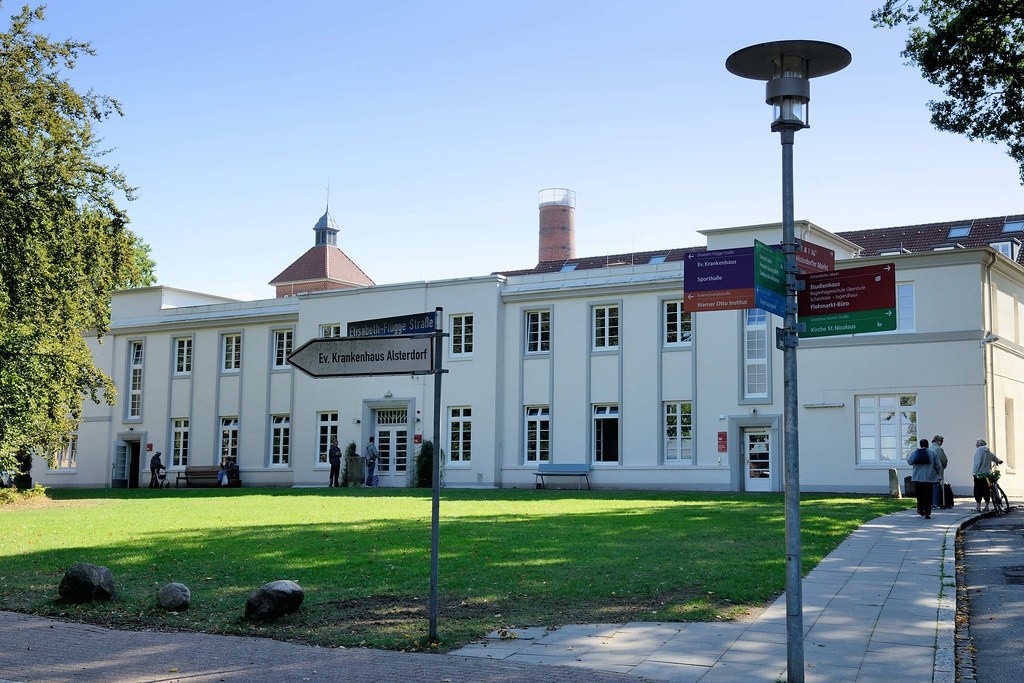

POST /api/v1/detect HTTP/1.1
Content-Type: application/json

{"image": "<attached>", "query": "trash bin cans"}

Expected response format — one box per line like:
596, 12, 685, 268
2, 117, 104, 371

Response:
904, 476, 917, 497
347, 457, 366, 481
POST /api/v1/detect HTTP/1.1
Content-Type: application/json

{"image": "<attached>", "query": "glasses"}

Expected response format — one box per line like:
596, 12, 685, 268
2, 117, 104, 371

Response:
937, 439, 943, 442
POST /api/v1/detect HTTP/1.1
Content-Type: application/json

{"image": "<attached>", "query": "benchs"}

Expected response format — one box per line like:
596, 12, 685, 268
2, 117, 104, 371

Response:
176, 465, 242, 488
532, 464, 591, 491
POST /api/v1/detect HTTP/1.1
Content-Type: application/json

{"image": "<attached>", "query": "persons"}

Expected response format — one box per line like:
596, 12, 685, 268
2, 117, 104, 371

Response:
329, 440, 342, 488
364, 436, 383, 487
217, 455, 236, 483
929, 435, 948, 510
907, 439, 940, 519
148, 452, 164, 488
971, 439, 1001, 512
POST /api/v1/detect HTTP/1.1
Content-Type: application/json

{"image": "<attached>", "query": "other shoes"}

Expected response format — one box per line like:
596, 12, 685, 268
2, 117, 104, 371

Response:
334, 485, 340, 487
329, 485, 334, 488
971, 508, 981, 512
917, 510, 931, 520
982, 508, 989, 511
364, 484, 372, 488
931, 504, 940, 509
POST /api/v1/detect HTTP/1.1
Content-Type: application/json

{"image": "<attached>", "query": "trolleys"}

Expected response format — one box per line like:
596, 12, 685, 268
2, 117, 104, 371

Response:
153, 467, 169, 488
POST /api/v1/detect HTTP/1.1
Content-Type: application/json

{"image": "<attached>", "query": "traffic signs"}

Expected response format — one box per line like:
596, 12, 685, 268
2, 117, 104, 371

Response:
286, 338, 434, 380
683, 238, 897, 338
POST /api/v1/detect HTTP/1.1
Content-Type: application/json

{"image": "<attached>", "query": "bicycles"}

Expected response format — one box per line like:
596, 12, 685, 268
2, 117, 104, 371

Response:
987, 462, 1009, 516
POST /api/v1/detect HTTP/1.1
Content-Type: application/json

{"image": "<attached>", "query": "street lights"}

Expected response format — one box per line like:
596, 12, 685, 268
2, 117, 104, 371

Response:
724, 40, 854, 683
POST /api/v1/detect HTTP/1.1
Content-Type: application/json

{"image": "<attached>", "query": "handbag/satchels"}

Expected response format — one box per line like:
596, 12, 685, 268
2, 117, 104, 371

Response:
914, 447, 932, 465
221, 473, 228, 486
369, 457, 375, 464
